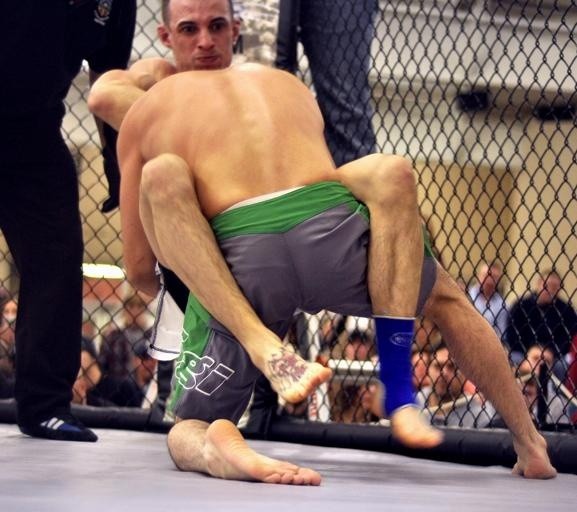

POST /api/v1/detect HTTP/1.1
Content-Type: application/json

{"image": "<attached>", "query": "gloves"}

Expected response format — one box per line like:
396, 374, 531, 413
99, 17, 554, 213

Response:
101, 159, 121, 212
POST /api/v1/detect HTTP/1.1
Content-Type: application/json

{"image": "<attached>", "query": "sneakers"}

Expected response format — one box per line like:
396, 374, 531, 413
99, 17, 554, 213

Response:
20, 414, 98, 442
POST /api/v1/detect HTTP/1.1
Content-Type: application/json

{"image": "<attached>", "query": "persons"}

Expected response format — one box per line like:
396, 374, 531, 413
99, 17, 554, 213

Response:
0, 296, 18, 383
294, 2, 379, 168
87, 1, 443, 448
411, 262, 576, 431
0, 1, 136, 442
116, 66, 556, 489
250, 309, 381, 424
72, 292, 156, 408
